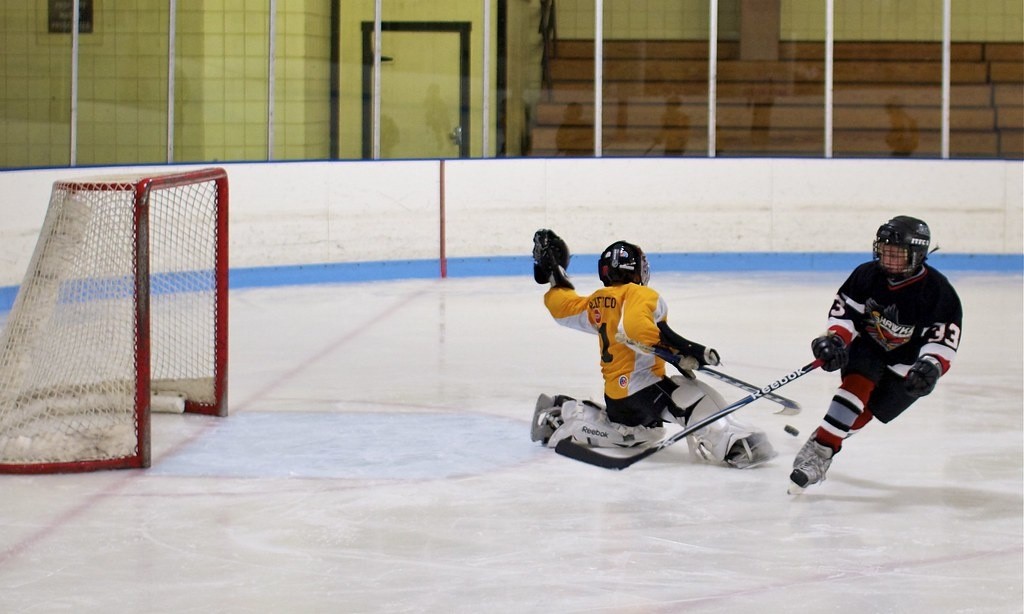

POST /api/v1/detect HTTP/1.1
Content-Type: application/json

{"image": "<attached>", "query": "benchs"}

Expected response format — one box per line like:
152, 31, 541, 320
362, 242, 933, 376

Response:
523, 39, 1024, 159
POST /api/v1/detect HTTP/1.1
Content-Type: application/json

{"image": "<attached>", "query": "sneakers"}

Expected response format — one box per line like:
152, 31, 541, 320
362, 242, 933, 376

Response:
786, 430, 850, 495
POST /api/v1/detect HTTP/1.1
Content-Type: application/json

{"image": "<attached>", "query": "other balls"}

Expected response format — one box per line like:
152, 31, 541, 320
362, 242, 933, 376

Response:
783, 423, 800, 436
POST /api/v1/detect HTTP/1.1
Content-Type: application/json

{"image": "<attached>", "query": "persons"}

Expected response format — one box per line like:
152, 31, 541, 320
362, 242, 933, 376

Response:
786, 216, 963, 497
530, 229, 781, 471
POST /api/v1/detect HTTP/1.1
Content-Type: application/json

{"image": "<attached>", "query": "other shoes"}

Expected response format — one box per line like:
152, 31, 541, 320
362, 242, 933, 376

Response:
530, 393, 557, 443
724, 433, 779, 469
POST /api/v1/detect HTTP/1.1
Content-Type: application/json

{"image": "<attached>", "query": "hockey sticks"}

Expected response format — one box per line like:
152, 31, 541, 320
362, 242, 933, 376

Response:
613, 333, 802, 417
554, 358, 827, 472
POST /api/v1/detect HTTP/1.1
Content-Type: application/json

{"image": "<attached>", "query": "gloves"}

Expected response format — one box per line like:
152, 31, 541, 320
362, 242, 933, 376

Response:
811, 336, 849, 372
902, 354, 942, 397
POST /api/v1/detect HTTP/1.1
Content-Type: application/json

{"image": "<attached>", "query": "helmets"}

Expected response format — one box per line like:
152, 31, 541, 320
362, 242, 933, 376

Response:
598, 241, 641, 286
877, 215, 930, 265
